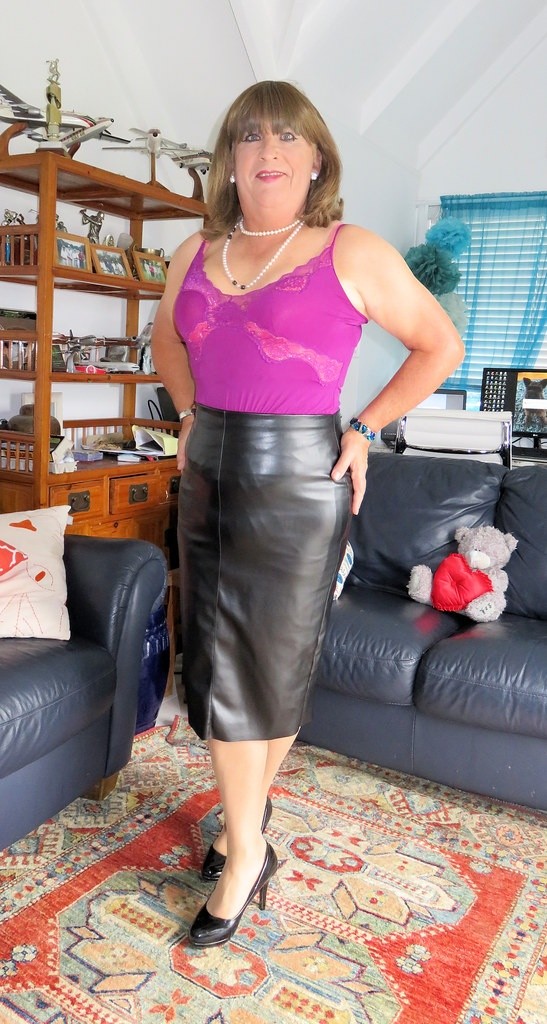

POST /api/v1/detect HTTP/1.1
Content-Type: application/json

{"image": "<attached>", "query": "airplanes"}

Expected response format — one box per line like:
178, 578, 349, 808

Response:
104, 321, 155, 348
129, 127, 214, 175
55, 330, 120, 352
0, 83, 131, 150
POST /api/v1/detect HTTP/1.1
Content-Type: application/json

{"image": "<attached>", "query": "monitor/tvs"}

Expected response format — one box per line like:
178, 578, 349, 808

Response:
416, 390, 467, 409
479, 367, 547, 458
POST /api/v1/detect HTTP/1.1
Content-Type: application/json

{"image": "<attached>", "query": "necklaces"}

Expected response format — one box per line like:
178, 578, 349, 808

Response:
222, 214, 306, 292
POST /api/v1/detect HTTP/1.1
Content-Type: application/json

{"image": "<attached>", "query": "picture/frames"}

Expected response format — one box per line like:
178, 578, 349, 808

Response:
130, 251, 167, 283
89, 245, 133, 277
53, 230, 92, 273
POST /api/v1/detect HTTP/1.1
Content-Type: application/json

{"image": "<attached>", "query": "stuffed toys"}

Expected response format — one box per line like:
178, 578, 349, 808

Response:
406, 526, 519, 622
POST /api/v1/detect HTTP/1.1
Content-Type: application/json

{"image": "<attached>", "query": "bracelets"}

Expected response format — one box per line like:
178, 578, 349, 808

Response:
179, 404, 197, 422
350, 418, 376, 443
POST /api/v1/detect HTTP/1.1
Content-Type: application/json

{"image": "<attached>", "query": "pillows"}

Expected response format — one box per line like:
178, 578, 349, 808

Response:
0, 505, 74, 640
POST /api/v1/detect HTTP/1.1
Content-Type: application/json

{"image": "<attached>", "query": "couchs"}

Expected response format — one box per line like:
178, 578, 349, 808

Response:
294, 452, 547, 811
0, 534, 167, 851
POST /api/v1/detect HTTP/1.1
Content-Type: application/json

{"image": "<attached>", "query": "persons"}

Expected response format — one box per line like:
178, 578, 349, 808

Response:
46, 58, 60, 82
1, 209, 26, 226
141, 260, 161, 280
56, 215, 67, 232
61, 245, 85, 268
151, 80, 465, 946
80, 209, 104, 244
97, 253, 126, 276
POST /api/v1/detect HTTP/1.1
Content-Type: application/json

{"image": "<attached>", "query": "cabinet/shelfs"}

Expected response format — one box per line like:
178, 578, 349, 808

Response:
0, 121, 213, 572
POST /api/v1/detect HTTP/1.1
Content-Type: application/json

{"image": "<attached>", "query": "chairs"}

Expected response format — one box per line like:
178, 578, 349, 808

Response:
393, 411, 513, 471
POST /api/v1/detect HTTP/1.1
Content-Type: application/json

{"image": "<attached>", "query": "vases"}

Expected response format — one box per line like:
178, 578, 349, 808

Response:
134, 605, 171, 734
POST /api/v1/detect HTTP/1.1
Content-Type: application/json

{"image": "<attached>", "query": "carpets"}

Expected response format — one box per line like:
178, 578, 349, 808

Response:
0, 715, 547, 1024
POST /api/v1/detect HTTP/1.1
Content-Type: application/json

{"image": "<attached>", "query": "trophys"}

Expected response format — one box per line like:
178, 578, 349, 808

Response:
132, 244, 165, 280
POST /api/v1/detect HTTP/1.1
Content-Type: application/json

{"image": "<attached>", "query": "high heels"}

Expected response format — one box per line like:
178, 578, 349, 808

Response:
201, 797, 272, 882
189, 844, 278, 947
